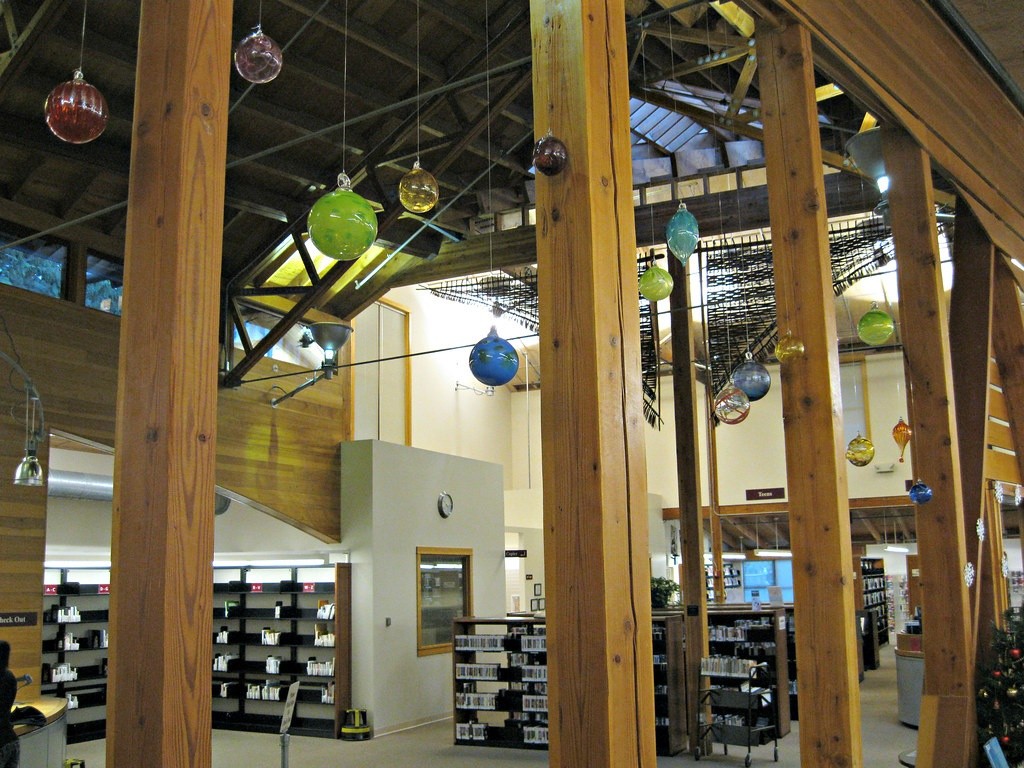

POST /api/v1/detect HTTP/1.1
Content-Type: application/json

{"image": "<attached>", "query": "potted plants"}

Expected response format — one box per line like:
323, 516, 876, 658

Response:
649, 576, 681, 607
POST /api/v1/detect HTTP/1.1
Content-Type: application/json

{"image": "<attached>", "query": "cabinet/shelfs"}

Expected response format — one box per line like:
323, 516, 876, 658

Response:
908, 555, 920, 617
212, 559, 347, 737
452, 615, 554, 751
42, 563, 110, 745
853, 560, 889, 646
651, 601, 799, 756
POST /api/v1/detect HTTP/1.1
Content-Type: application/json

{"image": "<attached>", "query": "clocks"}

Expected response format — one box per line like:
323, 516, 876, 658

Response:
437, 490, 454, 520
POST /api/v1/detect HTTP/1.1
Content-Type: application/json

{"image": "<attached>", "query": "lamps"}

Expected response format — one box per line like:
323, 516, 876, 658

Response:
454, 380, 495, 397
843, 126, 893, 226
1, 352, 47, 488
265, 323, 354, 409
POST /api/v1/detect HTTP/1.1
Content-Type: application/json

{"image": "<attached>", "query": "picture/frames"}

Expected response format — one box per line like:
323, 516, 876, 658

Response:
534, 583, 542, 596
538, 598, 545, 610
531, 599, 538, 610
